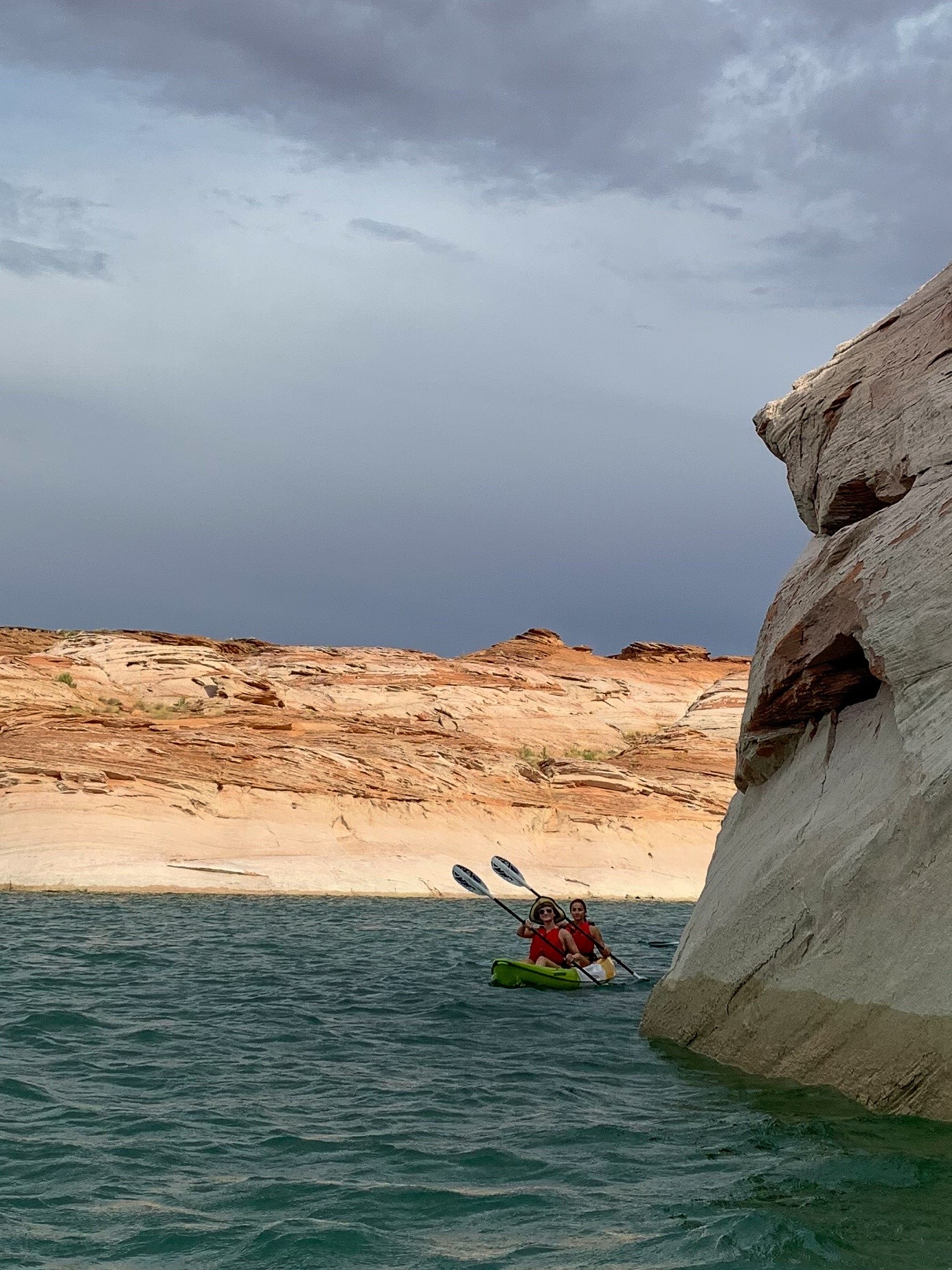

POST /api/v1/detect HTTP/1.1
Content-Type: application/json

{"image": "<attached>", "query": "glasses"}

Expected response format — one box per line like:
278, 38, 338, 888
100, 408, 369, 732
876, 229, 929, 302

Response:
539, 909, 552, 914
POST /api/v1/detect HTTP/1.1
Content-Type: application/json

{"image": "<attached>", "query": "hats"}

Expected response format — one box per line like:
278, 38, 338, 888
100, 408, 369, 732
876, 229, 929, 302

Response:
529, 896, 566, 925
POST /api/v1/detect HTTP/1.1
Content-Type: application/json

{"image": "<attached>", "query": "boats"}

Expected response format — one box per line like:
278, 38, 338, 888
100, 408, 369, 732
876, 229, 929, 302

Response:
491, 954, 617, 992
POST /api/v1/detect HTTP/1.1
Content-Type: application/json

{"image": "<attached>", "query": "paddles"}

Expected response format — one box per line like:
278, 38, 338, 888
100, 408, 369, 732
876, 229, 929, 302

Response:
637, 941, 680, 948
490, 856, 648, 980
452, 864, 606, 986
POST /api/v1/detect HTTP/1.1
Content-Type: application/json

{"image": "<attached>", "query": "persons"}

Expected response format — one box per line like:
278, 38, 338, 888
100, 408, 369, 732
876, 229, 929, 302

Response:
517, 897, 612, 968
